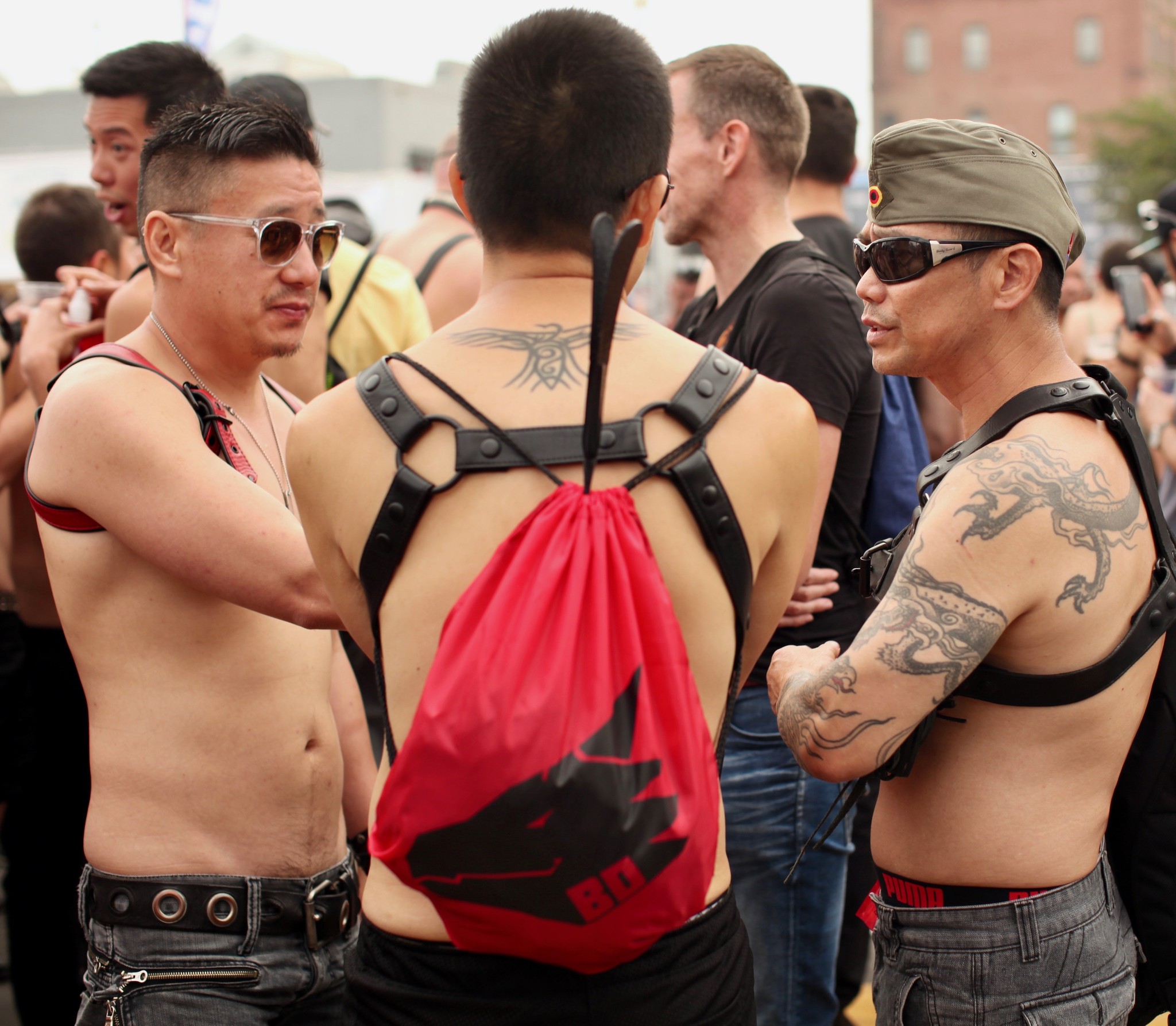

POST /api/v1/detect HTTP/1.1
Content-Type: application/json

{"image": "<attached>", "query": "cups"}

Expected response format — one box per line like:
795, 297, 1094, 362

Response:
14, 279, 77, 357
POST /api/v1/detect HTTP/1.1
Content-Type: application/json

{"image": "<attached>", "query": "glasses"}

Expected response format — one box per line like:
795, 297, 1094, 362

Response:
140, 212, 346, 271
621, 166, 675, 211
852, 236, 1043, 285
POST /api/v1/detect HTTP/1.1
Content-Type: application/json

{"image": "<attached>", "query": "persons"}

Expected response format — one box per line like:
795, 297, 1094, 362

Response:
0, 11, 1176, 1025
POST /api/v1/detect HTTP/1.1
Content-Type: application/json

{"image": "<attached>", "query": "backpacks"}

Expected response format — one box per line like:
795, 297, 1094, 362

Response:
368, 352, 761, 976
861, 374, 934, 551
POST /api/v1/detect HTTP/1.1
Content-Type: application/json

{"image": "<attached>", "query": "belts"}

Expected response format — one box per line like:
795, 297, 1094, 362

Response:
86, 844, 360, 954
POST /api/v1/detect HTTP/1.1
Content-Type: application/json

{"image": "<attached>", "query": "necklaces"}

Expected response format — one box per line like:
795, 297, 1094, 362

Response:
150, 314, 291, 510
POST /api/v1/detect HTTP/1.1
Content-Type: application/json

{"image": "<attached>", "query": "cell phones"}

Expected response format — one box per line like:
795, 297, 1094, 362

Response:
1111, 266, 1155, 335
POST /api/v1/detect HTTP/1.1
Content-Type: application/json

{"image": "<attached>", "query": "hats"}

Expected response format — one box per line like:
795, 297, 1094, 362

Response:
866, 118, 1086, 280
